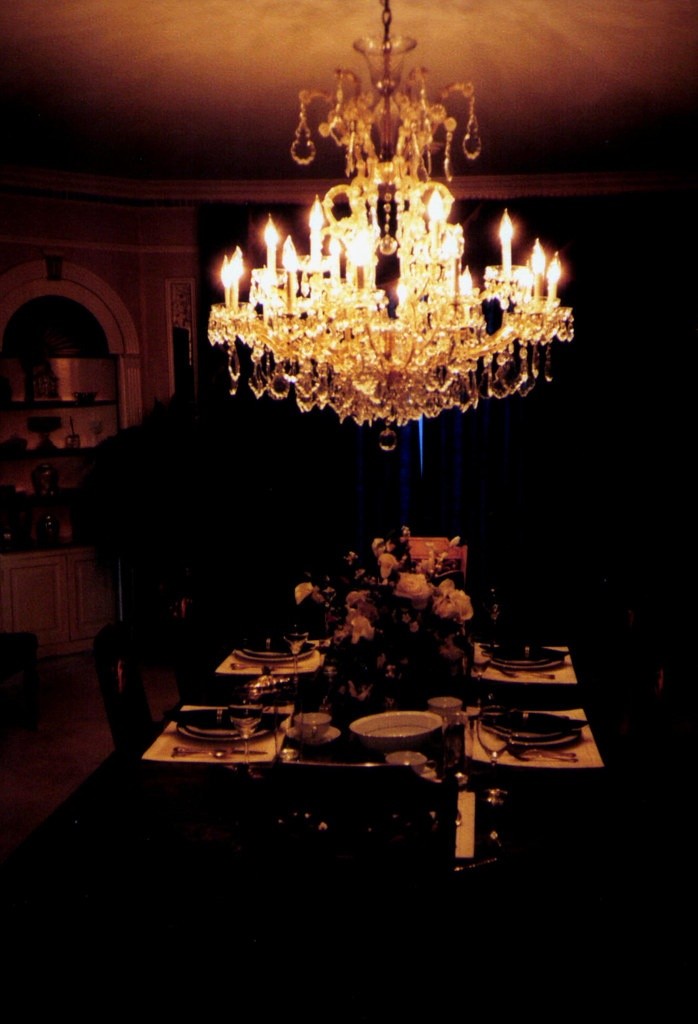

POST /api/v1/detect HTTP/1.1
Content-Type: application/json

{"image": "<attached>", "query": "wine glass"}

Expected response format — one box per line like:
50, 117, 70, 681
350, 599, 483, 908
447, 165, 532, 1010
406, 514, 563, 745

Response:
478, 694, 515, 803
281, 604, 309, 694
228, 685, 263, 766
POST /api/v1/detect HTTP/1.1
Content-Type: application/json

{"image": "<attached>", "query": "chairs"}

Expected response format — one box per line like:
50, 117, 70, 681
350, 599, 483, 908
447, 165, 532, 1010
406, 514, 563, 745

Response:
94, 602, 221, 744
581, 602, 668, 829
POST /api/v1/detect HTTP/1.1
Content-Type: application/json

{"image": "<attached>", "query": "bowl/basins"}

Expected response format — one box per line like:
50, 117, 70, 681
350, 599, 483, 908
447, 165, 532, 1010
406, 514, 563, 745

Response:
349, 712, 443, 751
73, 392, 96, 401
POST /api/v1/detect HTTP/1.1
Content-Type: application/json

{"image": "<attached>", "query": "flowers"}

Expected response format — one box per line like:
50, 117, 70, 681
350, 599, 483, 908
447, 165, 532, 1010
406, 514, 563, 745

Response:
297, 525, 473, 701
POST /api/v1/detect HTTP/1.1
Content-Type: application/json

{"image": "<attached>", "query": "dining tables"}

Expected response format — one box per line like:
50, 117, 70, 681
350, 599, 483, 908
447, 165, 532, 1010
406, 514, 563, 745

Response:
140, 641, 615, 888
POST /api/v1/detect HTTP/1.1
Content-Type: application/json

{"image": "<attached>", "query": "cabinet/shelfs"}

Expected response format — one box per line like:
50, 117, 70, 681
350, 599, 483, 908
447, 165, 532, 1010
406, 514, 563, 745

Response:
0, 346, 118, 661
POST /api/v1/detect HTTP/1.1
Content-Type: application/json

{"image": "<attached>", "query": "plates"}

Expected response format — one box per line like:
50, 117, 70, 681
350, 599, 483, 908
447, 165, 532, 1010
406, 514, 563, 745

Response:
284, 726, 341, 743
480, 718, 581, 746
176, 708, 269, 741
489, 651, 564, 669
233, 641, 315, 662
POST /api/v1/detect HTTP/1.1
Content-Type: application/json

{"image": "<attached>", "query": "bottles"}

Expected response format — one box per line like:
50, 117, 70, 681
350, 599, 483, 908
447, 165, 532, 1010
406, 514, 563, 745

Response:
424, 696, 463, 767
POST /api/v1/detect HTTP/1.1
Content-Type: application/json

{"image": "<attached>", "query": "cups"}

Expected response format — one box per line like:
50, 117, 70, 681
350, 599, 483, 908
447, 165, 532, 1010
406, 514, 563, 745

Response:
386, 751, 437, 775
294, 712, 332, 736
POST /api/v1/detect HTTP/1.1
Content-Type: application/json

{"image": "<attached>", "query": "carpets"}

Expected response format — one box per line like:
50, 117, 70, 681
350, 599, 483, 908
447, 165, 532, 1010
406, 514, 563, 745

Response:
0, 721, 698, 1024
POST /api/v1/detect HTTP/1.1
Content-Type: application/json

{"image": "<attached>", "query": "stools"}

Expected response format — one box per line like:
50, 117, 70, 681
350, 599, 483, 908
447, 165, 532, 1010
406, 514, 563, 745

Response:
0, 629, 38, 742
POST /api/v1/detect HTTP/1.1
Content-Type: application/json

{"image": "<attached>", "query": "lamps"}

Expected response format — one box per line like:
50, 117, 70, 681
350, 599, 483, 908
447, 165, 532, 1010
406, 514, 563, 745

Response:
207, 0, 575, 452
